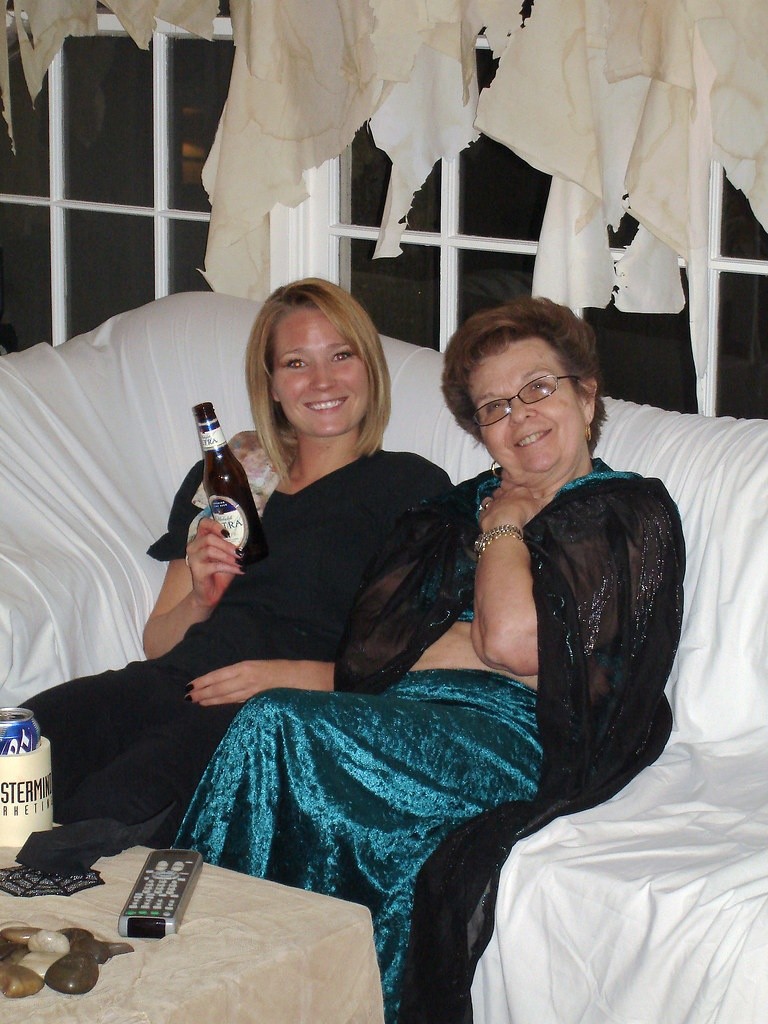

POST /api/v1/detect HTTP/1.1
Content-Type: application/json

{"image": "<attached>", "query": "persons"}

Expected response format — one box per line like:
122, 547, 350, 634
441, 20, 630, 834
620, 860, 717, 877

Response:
0, 278, 455, 849
172, 298, 686, 1024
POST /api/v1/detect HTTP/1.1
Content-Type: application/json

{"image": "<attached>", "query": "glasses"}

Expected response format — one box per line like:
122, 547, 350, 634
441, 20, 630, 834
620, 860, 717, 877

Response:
473, 374, 581, 426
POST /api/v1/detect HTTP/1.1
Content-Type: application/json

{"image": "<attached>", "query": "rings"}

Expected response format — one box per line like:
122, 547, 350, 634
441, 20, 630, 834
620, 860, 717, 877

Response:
484, 500, 491, 508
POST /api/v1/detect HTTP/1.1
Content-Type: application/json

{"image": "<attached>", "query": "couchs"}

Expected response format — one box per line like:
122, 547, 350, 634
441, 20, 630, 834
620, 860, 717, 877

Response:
0, 293, 767, 1024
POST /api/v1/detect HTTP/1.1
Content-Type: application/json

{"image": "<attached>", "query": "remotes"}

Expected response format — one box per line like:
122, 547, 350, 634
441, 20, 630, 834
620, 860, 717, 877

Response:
117, 848, 203, 940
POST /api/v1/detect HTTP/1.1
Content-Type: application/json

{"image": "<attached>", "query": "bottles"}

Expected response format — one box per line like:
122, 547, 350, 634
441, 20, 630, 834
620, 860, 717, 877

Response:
192, 402, 271, 568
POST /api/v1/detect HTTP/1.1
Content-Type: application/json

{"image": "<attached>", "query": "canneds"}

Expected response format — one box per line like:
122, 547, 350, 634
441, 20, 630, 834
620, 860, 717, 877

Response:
0, 707, 41, 754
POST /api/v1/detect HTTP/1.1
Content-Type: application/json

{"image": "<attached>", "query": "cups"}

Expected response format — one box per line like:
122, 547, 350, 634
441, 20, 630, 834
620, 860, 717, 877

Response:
0, 736, 52, 848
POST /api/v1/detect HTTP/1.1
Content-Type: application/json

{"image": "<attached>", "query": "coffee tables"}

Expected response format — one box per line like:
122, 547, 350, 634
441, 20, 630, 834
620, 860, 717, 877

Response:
0, 821, 386, 1024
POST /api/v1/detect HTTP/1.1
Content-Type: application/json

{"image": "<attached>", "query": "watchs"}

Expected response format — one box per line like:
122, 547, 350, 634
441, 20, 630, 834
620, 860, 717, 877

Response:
473, 524, 522, 558
